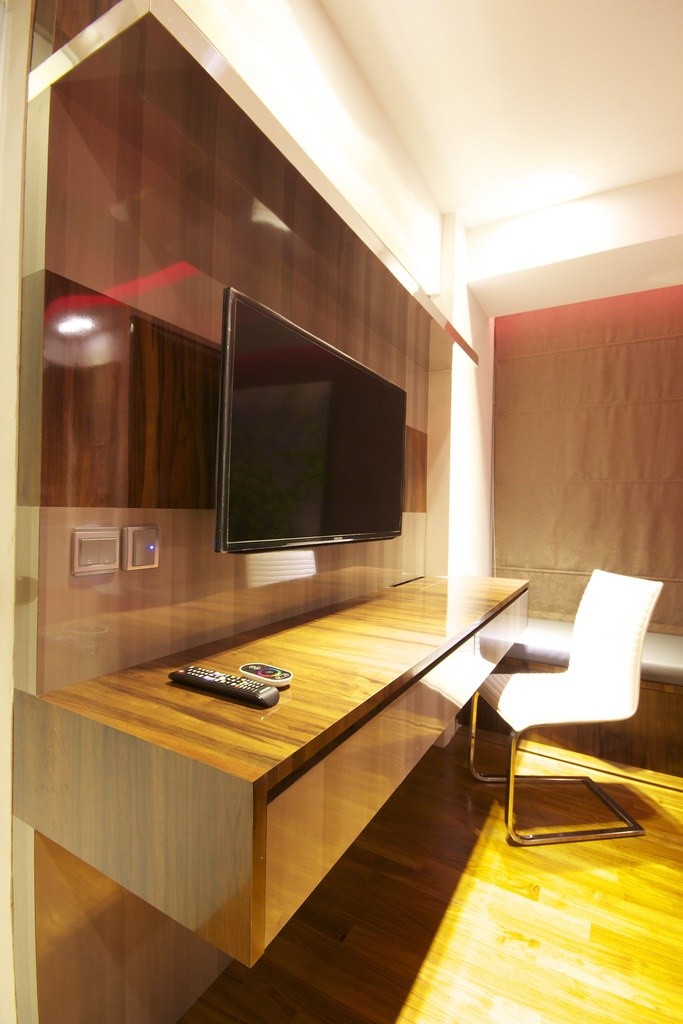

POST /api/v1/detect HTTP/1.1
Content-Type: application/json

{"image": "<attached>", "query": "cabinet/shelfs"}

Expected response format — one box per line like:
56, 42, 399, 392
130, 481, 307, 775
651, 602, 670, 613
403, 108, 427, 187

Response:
28, 569, 533, 969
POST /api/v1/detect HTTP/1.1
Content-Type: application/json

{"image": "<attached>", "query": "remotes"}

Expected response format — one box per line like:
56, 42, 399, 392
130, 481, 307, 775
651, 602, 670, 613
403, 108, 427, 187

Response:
169, 665, 280, 708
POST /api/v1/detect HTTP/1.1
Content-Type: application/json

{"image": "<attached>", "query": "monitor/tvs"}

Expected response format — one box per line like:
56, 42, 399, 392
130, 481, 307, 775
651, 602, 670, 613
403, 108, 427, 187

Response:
215, 287, 408, 553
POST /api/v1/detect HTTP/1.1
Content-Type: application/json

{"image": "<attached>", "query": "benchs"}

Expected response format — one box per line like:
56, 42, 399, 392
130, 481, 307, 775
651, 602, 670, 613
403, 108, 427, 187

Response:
460, 604, 683, 775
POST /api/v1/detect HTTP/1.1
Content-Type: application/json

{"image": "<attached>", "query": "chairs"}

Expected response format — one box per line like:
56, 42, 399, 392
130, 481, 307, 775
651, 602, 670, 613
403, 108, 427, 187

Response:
467, 570, 664, 844
